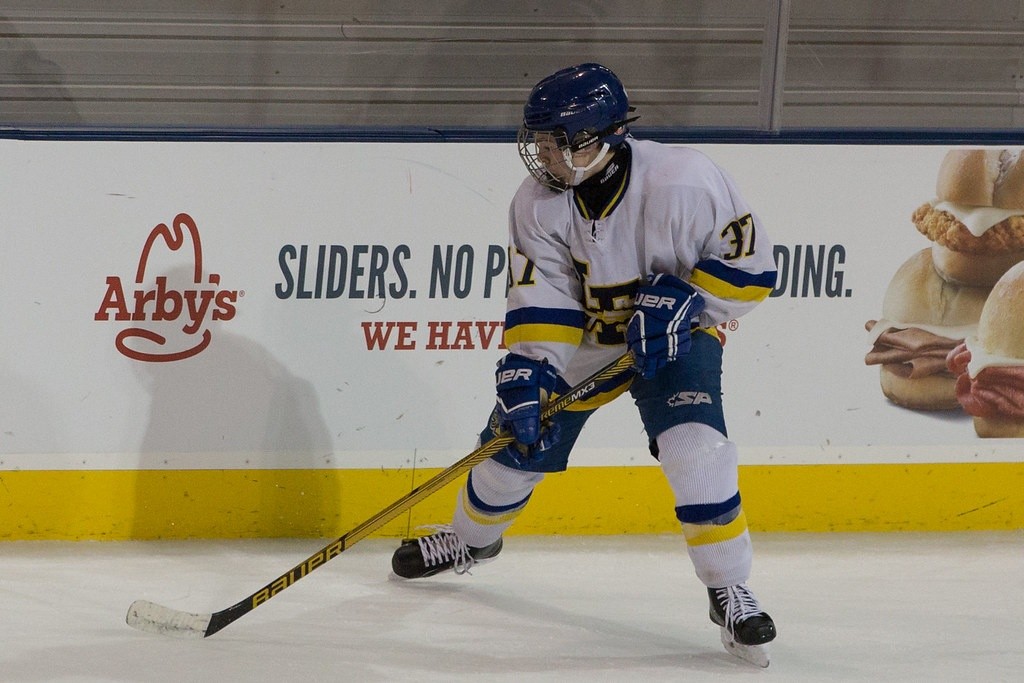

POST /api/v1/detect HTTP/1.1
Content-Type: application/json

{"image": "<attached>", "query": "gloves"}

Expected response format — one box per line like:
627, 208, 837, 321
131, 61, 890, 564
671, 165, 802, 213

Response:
624, 273, 705, 379
486, 352, 558, 464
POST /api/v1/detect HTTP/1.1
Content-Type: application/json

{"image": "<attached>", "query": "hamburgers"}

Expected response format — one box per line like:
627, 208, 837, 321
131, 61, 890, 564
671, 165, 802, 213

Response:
864, 144, 1024, 438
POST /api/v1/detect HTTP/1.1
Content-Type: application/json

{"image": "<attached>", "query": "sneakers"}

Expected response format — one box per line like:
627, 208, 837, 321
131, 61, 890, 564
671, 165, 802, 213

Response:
706, 583, 777, 667
389, 530, 503, 583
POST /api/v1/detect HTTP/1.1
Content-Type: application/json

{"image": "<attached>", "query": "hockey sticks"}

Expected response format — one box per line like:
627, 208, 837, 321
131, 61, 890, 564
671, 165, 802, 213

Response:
127, 320, 703, 639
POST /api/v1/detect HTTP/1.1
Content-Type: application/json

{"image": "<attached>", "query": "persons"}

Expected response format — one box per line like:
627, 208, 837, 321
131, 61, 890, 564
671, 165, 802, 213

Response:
392, 63, 778, 668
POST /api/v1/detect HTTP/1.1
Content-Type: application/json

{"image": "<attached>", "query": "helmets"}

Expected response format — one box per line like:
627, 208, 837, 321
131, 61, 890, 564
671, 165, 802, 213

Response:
524, 63, 630, 151
